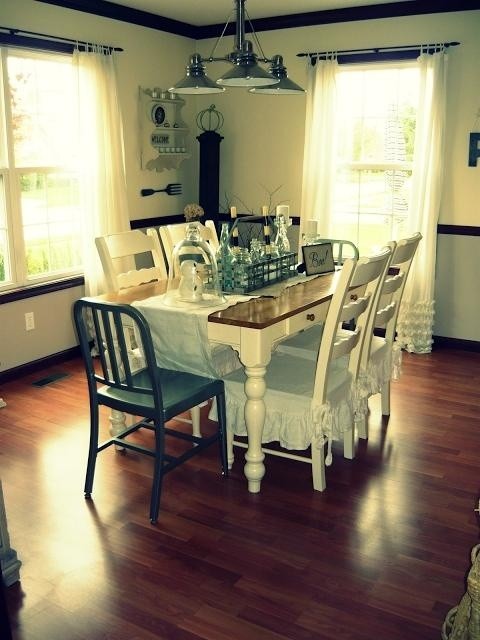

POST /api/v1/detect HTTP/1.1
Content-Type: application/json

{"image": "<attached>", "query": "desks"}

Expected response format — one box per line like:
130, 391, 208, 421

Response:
83, 256, 389, 493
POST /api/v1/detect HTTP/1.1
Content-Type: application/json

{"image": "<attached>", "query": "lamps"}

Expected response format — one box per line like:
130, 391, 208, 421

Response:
167, 0, 307, 95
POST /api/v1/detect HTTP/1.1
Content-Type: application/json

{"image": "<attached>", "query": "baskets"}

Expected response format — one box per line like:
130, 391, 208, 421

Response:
439, 544, 480, 640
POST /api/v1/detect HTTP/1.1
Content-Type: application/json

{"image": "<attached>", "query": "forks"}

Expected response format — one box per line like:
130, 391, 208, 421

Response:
141, 183, 182, 196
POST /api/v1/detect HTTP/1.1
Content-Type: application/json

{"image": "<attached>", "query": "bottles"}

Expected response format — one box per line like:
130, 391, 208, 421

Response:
213, 205, 290, 293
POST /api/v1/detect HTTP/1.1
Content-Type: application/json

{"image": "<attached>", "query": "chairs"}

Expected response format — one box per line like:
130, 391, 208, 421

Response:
70, 296, 230, 522
93, 227, 217, 449
223, 246, 392, 492
277, 232, 423, 440
161, 223, 361, 266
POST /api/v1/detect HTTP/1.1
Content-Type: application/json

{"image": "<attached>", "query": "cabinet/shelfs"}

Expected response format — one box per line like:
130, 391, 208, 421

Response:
141, 86, 193, 171
